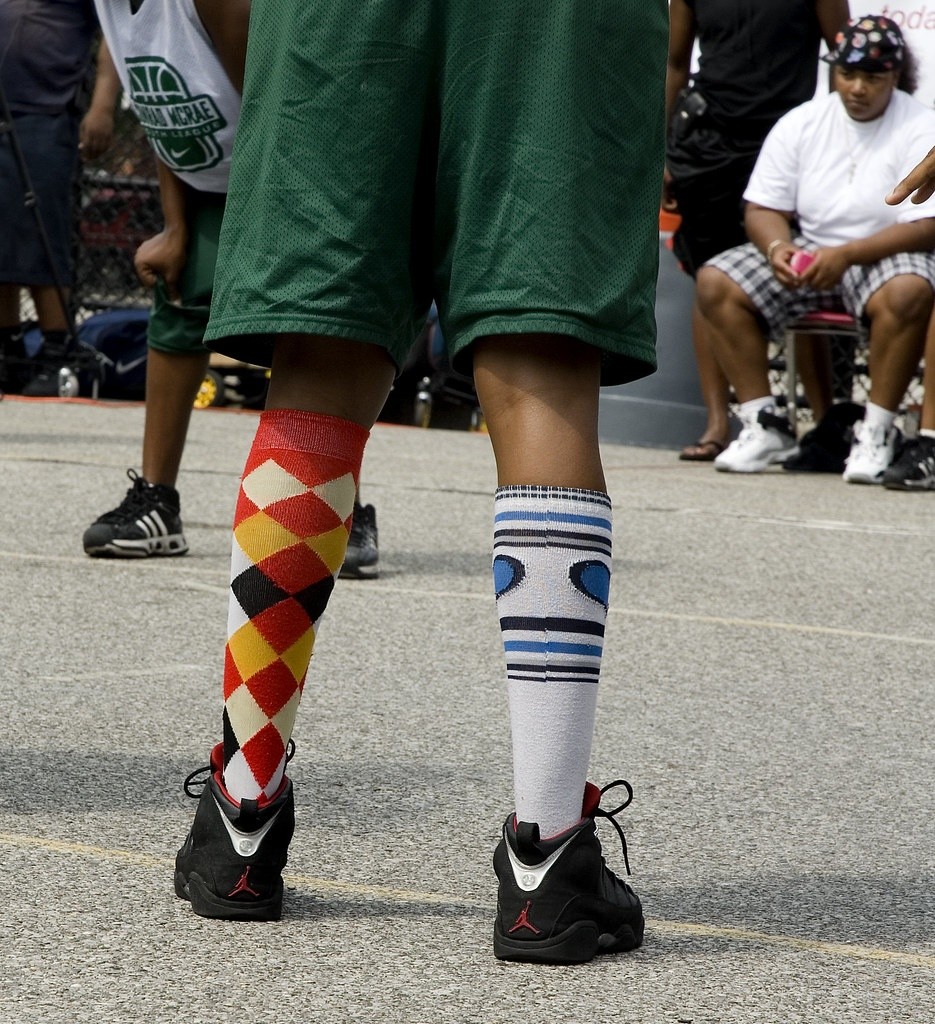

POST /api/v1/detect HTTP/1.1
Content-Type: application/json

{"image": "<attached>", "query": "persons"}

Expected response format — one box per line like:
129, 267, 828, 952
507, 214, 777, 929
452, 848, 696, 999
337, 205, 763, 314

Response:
177, 0, 674, 964
82, 0, 382, 581
664, 0, 935, 492
0, 0, 121, 398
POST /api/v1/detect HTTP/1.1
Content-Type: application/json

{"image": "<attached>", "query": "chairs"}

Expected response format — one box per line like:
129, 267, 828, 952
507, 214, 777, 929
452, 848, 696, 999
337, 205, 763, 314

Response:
782, 309, 863, 437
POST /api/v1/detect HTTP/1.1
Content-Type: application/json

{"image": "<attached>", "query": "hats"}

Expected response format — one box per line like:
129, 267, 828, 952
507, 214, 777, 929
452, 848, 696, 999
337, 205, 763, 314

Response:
821, 15, 906, 73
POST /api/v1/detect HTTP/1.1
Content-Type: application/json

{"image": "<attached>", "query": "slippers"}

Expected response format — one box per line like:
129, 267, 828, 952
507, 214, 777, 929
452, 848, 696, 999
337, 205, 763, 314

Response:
679, 440, 723, 462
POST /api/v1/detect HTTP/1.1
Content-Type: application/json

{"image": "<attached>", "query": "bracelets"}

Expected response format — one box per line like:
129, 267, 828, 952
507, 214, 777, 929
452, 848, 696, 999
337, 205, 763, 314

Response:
766, 239, 788, 265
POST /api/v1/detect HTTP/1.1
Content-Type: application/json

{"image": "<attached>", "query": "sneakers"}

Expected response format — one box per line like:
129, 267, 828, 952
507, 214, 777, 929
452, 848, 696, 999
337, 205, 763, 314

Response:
82, 478, 189, 558
884, 437, 935, 491
490, 783, 648, 962
175, 743, 296, 922
842, 419, 896, 484
339, 501, 379, 579
713, 397, 801, 473
0, 327, 84, 396
